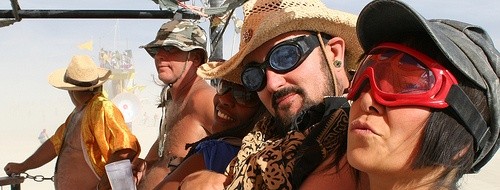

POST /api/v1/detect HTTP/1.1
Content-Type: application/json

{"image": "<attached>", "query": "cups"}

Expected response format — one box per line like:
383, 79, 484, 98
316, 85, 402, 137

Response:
105, 159, 137, 190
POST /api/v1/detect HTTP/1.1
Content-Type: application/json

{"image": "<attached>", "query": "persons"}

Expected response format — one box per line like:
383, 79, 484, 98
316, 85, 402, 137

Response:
347, 0, 500, 190
196, 0, 359, 190
4, 55, 141, 190
136, 20, 219, 190
210, 79, 266, 137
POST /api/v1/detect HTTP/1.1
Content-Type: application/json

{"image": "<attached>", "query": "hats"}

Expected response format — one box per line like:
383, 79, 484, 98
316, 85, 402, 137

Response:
196, 1, 365, 81
356, 0, 500, 174
138, 19, 208, 55
47, 54, 112, 91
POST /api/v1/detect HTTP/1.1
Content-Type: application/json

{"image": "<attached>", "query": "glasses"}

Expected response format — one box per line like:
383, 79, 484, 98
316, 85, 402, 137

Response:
216, 77, 255, 105
240, 33, 332, 92
152, 46, 173, 52
347, 42, 493, 152
